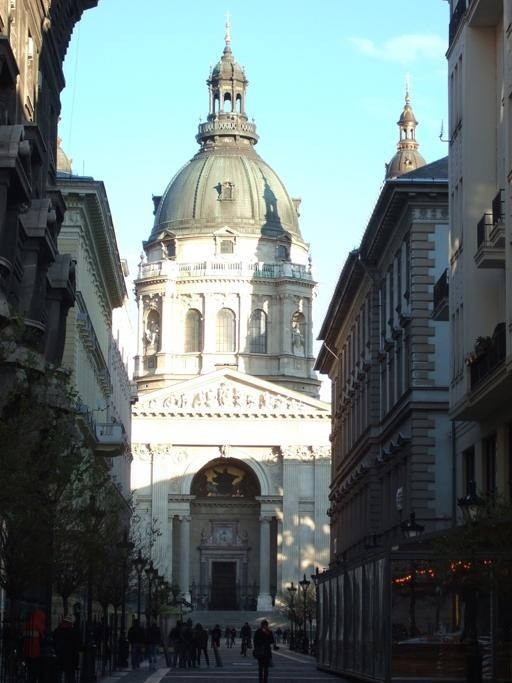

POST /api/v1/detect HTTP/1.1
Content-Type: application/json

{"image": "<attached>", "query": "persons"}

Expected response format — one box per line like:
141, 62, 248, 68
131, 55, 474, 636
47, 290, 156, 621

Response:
24, 604, 124, 682
127, 615, 290, 672
252, 619, 277, 683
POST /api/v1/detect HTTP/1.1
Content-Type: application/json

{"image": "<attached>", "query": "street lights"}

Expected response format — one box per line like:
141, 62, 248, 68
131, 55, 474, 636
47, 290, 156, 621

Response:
310, 565, 323, 652
400, 510, 426, 638
456, 478, 487, 640
364, 533, 386, 642
78, 492, 107, 681
116, 528, 137, 668
132, 547, 180, 664
299, 573, 310, 654
37, 457, 70, 681
287, 581, 297, 649
